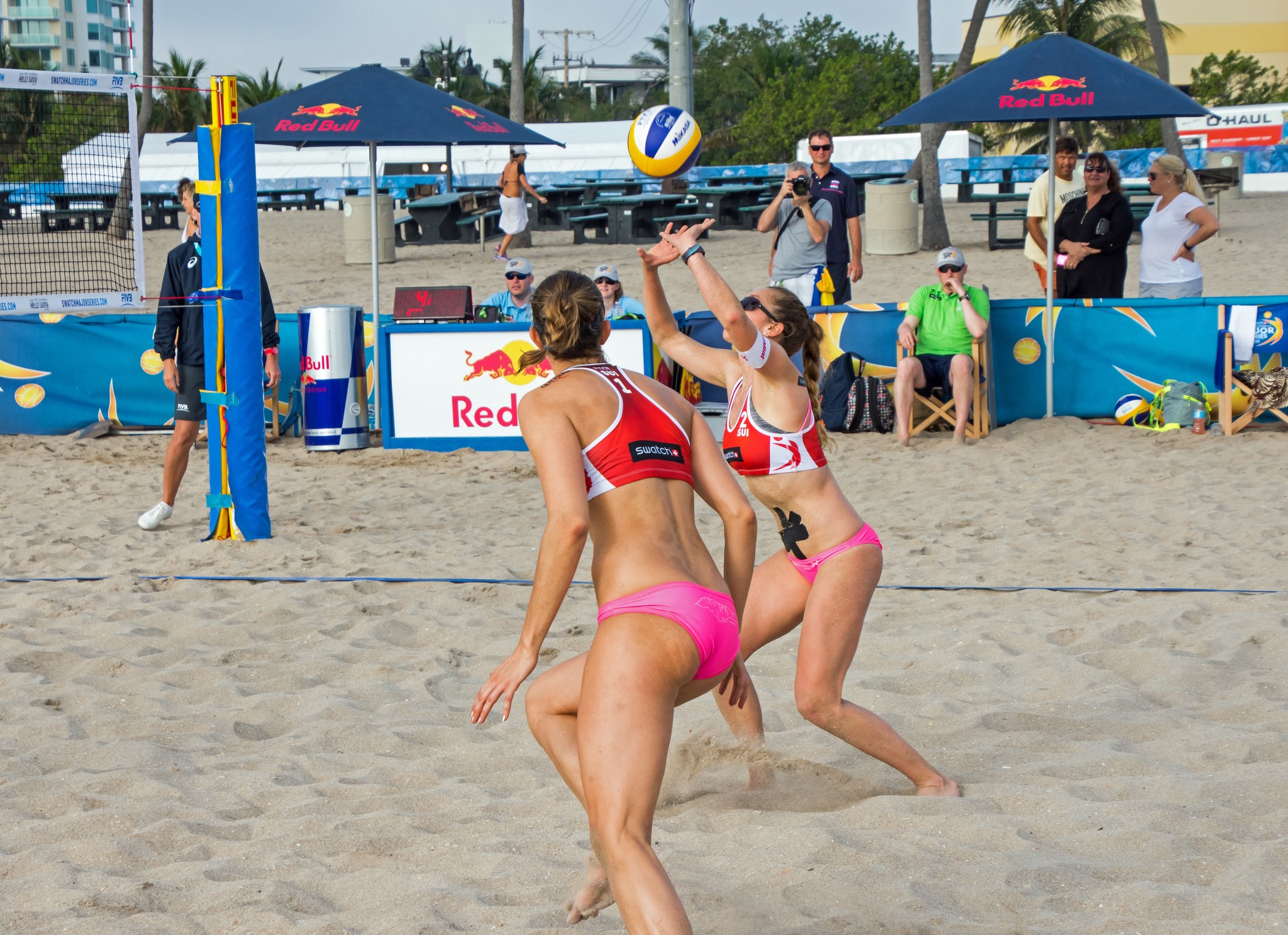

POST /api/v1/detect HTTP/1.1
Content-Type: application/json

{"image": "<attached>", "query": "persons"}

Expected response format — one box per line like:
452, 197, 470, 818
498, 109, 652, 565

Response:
493, 145, 547, 261
593, 264, 645, 320
480, 257, 536, 322
177, 177, 196, 243
808, 129, 864, 305
470, 270, 756, 935
1137, 154, 1219, 298
1023, 137, 1087, 299
758, 160, 835, 306
1053, 152, 1134, 299
137, 191, 281, 531
636, 217, 957, 799
893, 246, 990, 446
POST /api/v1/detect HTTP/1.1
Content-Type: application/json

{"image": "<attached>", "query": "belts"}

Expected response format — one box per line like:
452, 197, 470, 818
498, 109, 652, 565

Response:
1096, 218, 1111, 235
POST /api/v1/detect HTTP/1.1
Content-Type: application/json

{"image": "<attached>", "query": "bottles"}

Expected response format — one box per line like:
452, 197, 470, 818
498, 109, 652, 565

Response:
1211, 422, 1223, 436
612, 301, 622, 318
1193, 402, 1206, 434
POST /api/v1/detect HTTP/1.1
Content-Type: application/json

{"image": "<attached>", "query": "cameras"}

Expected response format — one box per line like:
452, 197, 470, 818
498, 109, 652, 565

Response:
787, 174, 810, 196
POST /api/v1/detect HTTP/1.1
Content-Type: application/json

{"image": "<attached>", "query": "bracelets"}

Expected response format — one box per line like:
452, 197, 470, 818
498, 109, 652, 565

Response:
681, 244, 705, 265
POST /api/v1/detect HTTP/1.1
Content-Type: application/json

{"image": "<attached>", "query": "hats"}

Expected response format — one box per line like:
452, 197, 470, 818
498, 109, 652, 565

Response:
512, 145, 530, 155
505, 257, 533, 275
593, 264, 619, 280
937, 246, 965, 268
192, 192, 200, 206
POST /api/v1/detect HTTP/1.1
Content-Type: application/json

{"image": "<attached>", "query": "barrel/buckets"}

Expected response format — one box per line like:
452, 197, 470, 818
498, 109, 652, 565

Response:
342, 194, 396, 264
864, 177, 919, 254
1207, 151, 1244, 199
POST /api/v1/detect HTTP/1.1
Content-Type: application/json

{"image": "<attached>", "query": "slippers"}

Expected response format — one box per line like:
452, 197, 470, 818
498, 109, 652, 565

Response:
923, 423, 955, 432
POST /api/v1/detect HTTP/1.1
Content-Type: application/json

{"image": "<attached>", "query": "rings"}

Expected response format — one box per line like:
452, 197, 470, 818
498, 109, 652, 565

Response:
1070, 263, 1074, 266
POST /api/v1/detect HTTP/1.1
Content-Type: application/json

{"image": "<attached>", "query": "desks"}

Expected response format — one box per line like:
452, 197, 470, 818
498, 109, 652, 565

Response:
952, 164, 1052, 200
47, 192, 121, 227
258, 188, 322, 211
133, 192, 175, 229
706, 173, 785, 186
575, 177, 655, 182
550, 182, 663, 199
595, 192, 686, 241
970, 191, 1030, 249
1119, 183, 1159, 204
337, 186, 412, 197
524, 188, 589, 228
403, 191, 489, 243
379, 310, 685, 453
0, 189, 18, 219
686, 185, 769, 231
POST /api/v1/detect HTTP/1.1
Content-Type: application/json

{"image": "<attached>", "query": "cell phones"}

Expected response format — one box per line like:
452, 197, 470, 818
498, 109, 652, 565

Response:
1056, 254, 1068, 266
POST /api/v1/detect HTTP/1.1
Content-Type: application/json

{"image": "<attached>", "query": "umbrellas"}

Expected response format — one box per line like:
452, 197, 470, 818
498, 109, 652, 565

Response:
165, 64, 566, 429
876, 31, 1220, 419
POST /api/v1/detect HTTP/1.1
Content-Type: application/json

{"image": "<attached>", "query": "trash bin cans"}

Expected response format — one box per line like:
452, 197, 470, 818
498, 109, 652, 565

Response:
862, 178, 920, 256
299, 305, 372, 451
341, 195, 396, 262
1206, 149, 1244, 198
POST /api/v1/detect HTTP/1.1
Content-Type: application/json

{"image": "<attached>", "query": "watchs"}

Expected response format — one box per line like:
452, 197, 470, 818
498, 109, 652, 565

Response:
1184, 240, 1194, 251
959, 293, 970, 302
264, 347, 278, 355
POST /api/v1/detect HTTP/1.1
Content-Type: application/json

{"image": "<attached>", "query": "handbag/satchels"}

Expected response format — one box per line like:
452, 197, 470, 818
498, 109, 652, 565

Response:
612, 312, 646, 320
471, 304, 506, 323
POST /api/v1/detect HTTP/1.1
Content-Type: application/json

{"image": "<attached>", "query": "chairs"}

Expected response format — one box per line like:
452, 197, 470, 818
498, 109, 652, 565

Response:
194, 319, 278, 446
896, 326, 988, 440
1217, 302, 1288, 436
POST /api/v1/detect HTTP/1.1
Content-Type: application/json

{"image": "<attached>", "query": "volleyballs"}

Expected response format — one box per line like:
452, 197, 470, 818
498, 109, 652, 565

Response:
1150, 398, 1165, 426
627, 104, 703, 179
1115, 394, 1150, 426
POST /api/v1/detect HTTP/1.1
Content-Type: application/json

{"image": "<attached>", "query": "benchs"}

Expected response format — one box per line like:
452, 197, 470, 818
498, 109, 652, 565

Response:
0, 185, 907, 243
947, 178, 1216, 249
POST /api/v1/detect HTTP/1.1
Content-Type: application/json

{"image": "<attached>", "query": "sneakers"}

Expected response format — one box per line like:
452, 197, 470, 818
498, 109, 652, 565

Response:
138, 501, 175, 530
494, 251, 512, 262
495, 243, 508, 258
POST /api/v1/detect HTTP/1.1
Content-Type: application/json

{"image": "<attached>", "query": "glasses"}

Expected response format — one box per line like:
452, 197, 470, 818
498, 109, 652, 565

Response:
939, 263, 964, 273
594, 277, 619, 285
809, 144, 832, 151
504, 273, 530, 280
522, 153, 527, 159
1084, 165, 1108, 173
193, 205, 200, 213
1148, 172, 1169, 180
739, 296, 789, 341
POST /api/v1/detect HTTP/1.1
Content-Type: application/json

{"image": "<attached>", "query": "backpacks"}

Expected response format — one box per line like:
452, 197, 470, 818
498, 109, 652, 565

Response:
817, 351, 866, 431
1132, 378, 1212, 433
841, 375, 895, 434
